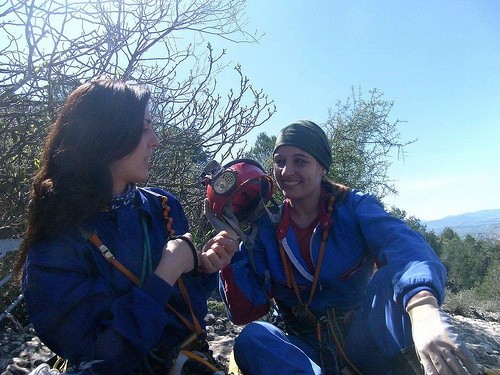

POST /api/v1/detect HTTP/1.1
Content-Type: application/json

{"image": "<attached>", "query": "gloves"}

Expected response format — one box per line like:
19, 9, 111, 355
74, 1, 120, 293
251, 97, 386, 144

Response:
204, 198, 239, 253
406, 295, 472, 375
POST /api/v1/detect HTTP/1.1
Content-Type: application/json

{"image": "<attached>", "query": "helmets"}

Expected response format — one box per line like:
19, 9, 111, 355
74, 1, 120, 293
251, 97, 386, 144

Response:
207, 159, 274, 222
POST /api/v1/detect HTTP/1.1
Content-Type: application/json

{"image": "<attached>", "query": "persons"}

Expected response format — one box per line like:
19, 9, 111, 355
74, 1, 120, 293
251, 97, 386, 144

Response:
218, 119, 482, 375
20, 79, 237, 375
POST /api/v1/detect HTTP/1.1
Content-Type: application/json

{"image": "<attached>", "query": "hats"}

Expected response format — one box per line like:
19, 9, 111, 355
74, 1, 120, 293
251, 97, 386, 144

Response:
273, 120, 332, 174
